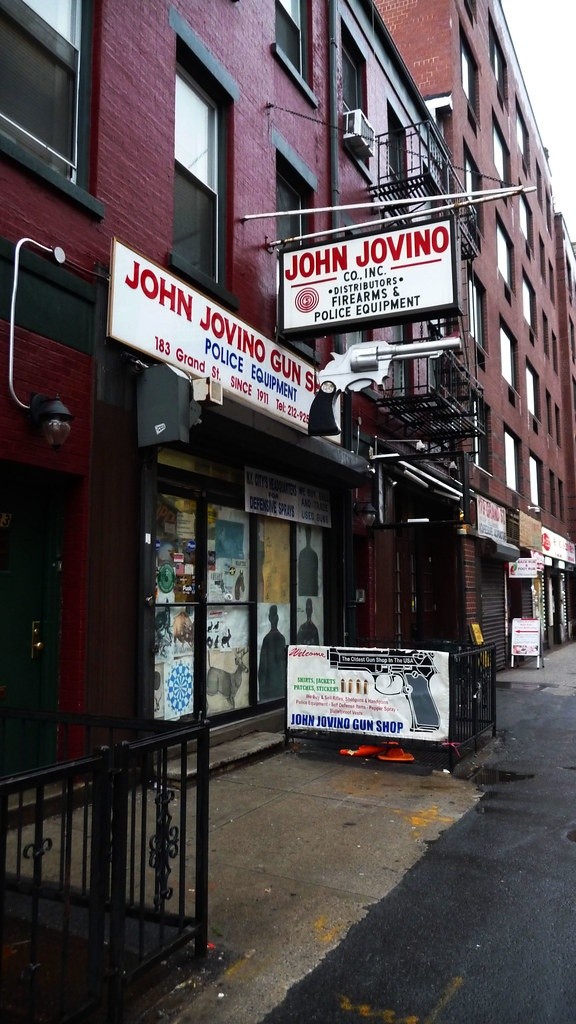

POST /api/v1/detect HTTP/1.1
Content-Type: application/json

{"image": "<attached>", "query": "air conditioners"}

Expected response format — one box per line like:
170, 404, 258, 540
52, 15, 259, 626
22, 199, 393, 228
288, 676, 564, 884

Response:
343, 108, 376, 159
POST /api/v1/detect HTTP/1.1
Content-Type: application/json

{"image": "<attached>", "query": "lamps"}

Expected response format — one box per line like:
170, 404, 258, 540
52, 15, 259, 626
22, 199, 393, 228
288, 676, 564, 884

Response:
382, 440, 427, 452
419, 460, 458, 472
354, 499, 377, 528
31, 390, 73, 452
528, 505, 541, 515
384, 475, 398, 490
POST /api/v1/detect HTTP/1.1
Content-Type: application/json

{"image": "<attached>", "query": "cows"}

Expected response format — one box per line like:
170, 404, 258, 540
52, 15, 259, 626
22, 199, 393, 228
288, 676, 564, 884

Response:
172, 612, 194, 648
